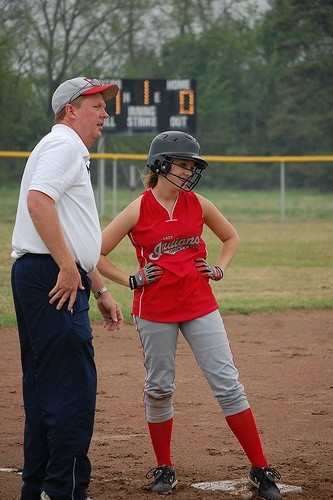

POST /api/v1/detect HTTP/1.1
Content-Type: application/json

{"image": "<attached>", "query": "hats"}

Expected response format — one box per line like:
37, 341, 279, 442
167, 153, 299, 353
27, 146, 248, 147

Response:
52, 76, 120, 115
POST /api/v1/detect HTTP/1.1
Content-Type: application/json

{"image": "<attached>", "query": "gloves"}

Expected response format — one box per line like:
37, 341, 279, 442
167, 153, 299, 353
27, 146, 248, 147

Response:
195, 258, 223, 281
129, 262, 164, 290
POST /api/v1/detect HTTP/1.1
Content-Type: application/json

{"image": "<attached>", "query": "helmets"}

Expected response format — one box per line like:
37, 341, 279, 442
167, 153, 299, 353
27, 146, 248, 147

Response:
148, 130, 208, 174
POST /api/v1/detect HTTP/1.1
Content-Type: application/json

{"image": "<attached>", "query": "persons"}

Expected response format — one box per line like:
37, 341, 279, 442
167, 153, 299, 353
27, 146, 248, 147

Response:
97, 130, 284, 500
12, 76, 124, 500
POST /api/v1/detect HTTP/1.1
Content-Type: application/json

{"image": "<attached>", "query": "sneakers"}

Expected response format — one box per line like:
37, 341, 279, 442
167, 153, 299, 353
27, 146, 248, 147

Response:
145, 464, 179, 497
248, 465, 284, 500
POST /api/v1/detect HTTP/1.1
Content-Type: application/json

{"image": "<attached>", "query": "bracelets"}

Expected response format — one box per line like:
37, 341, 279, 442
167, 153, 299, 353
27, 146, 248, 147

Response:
94, 287, 107, 299
